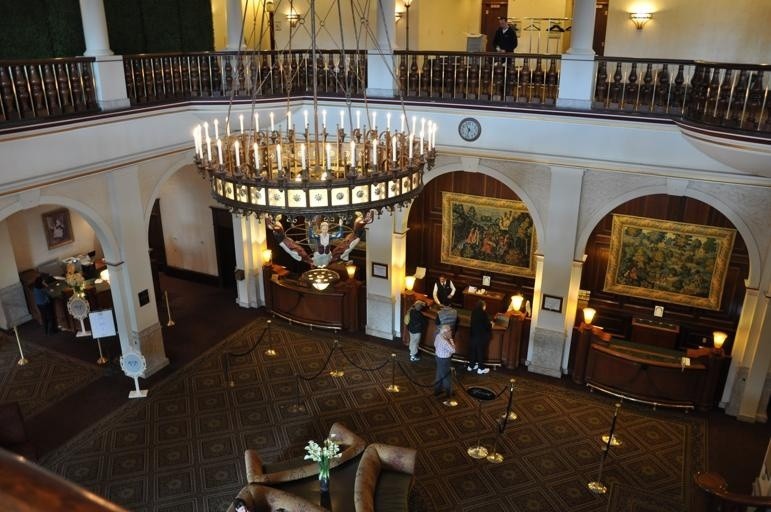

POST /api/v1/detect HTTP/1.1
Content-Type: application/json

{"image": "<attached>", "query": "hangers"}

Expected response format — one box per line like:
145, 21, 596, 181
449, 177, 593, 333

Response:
523, 23, 572, 31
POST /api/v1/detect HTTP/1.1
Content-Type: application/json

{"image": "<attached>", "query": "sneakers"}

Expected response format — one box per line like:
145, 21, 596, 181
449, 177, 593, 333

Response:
477, 368, 490, 374
467, 364, 478, 371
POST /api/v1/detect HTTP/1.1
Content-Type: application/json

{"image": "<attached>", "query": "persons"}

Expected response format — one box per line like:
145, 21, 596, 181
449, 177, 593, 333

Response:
265, 213, 315, 268
331, 209, 378, 263
505, 289, 533, 320
307, 217, 345, 269
433, 324, 457, 404
492, 15, 519, 100
404, 298, 428, 361
32, 275, 56, 338
432, 273, 457, 307
466, 299, 493, 375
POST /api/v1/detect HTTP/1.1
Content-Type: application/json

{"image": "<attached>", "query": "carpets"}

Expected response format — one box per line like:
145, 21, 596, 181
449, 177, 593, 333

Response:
37, 315, 710, 512
0, 331, 109, 423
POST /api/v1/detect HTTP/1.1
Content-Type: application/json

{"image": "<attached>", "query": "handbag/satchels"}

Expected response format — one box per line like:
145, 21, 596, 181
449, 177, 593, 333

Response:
404, 309, 410, 325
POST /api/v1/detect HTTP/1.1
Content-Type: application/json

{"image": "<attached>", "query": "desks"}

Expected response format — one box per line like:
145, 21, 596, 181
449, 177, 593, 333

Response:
76, 257, 106, 279
462, 286, 506, 313
625, 318, 680, 349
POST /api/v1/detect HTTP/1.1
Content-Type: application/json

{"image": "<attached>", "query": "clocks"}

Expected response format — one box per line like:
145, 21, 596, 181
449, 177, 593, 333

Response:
458, 118, 481, 141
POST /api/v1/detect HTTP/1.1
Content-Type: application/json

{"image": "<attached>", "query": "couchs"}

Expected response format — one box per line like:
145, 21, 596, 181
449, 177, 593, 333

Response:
226, 421, 417, 512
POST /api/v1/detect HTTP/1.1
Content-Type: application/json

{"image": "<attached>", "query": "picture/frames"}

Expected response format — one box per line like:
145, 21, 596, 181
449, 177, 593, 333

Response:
41, 208, 75, 250
440, 191, 538, 279
372, 262, 388, 279
542, 293, 563, 312
603, 214, 737, 312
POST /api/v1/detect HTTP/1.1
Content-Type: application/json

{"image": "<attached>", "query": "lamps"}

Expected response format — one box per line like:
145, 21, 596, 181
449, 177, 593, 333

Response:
629, 13, 652, 31
286, 15, 299, 27
511, 295, 523, 311
395, 0, 412, 97
193, 0, 436, 291
262, 249, 272, 264
583, 307, 597, 325
346, 266, 356, 279
405, 276, 416, 290
713, 331, 728, 349
